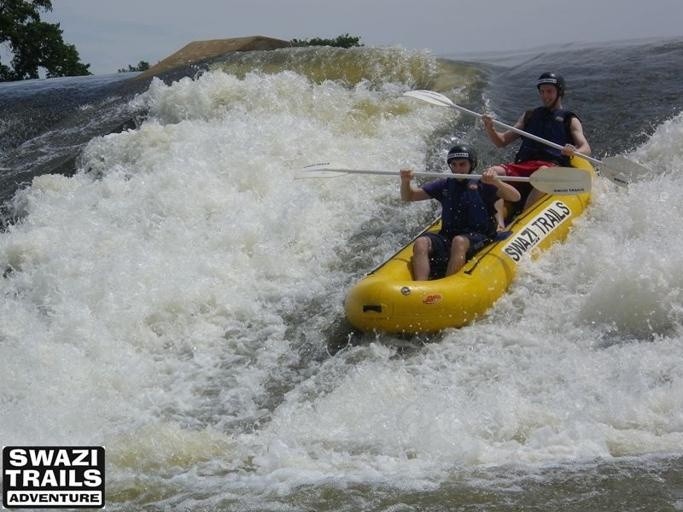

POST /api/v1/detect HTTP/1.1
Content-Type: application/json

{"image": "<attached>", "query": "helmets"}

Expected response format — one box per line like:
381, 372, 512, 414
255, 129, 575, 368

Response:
447, 144, 478, 169
536, 71, 566, 90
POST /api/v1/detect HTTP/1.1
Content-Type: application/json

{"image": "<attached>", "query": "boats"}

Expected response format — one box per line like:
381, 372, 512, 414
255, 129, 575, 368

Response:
342, 147, 596, 342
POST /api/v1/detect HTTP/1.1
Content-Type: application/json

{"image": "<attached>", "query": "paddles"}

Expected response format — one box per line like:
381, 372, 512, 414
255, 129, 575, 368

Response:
293, 160, 592, 195
402, 88, 654, 188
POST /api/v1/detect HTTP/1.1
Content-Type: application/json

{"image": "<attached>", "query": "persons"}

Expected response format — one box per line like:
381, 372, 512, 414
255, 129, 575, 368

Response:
479, 71, 592, 232
399, 143, 522, 281
